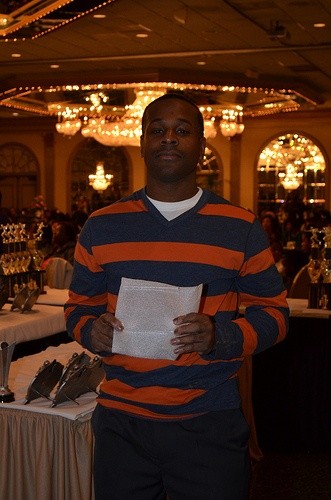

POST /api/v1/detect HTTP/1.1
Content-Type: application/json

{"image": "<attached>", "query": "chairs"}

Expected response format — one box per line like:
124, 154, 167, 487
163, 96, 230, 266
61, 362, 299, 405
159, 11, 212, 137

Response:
40, 258, 74, 289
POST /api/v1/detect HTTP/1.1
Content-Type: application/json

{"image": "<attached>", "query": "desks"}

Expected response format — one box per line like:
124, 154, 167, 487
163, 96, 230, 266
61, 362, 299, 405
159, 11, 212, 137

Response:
0, 286, 69, 350
0, 342, 103, 500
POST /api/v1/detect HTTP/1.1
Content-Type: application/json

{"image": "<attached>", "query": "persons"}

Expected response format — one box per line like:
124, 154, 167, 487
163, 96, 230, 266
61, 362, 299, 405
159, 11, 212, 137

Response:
0, 203, 299, 279
64, 94, 289, 500
